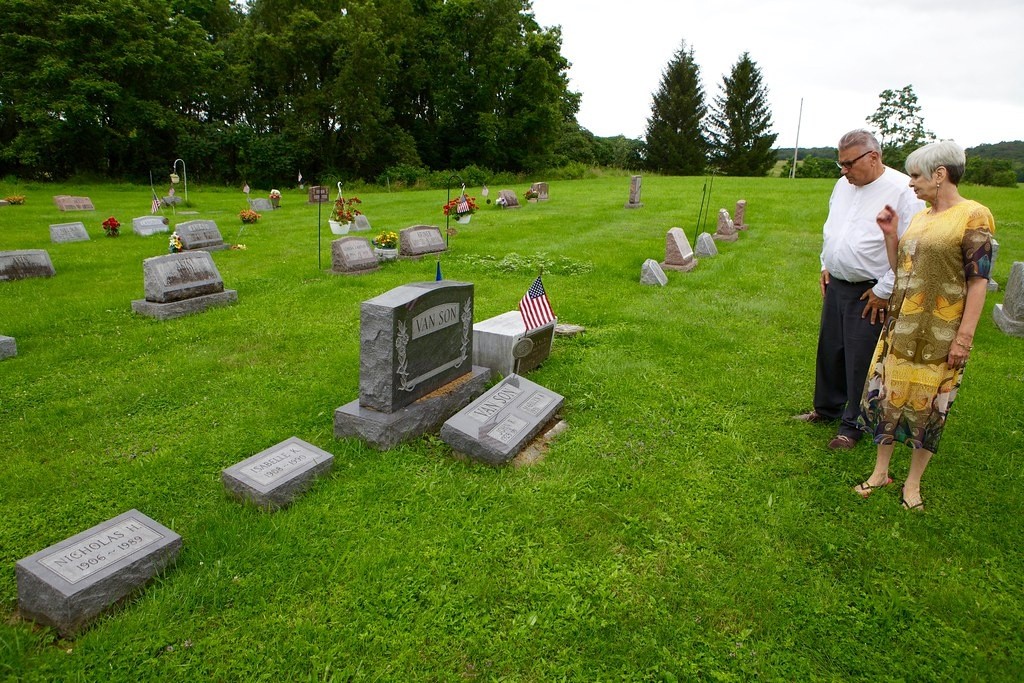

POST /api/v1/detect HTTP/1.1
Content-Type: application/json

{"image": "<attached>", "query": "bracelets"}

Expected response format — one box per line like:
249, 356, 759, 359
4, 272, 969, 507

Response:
955, 338, 974, 351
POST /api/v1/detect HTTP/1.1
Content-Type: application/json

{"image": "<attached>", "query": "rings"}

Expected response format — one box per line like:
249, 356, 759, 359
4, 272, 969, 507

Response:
879, 309, 884, 313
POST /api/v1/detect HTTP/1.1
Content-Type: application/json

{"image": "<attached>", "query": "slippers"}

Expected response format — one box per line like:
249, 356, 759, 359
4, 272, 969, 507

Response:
853, 477, 892, 499
902, 483, 924, 511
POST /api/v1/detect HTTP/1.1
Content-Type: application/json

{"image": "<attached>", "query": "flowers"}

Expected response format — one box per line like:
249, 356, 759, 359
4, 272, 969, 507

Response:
331, 195, 362, 225
442, 194, 481, 220
269, 194, 281, 198
522, 189, 538, 200
168, 234, 184, 252
496, 197, 509, 208
374, 231, 399, 250
238, 209, 261, 223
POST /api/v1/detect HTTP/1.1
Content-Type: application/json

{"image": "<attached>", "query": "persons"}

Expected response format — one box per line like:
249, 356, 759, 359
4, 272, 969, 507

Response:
854, 140, 996, 512
794, 129, 926, 450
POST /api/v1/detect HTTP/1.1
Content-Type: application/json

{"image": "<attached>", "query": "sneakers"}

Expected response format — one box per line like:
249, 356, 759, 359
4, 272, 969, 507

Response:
827, 435, 857, 450
794, 410, 824, 424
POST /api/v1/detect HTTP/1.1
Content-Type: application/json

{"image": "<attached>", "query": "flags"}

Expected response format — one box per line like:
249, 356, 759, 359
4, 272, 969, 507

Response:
457, 189, 469, 213
298, 171, 302, 181
151, 194, 161, 215
518, 275, 556, 331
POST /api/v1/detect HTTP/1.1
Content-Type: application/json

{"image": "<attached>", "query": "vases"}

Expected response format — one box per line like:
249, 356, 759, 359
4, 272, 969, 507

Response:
374, 248, 399, 261
270, 197, 280, 206
499, 204, 504, 209
242, 219, 255, 222
455, 213, 472, 224
527, 198, 538, 203
173, 247, 178, 252
328, 219, 351, 235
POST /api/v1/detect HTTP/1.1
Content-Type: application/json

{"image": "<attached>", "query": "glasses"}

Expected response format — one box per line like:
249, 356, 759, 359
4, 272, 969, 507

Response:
835, 151, 872, 169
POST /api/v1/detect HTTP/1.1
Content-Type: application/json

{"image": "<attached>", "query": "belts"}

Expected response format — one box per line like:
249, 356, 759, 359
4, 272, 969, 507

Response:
865, 280, 877, 285
